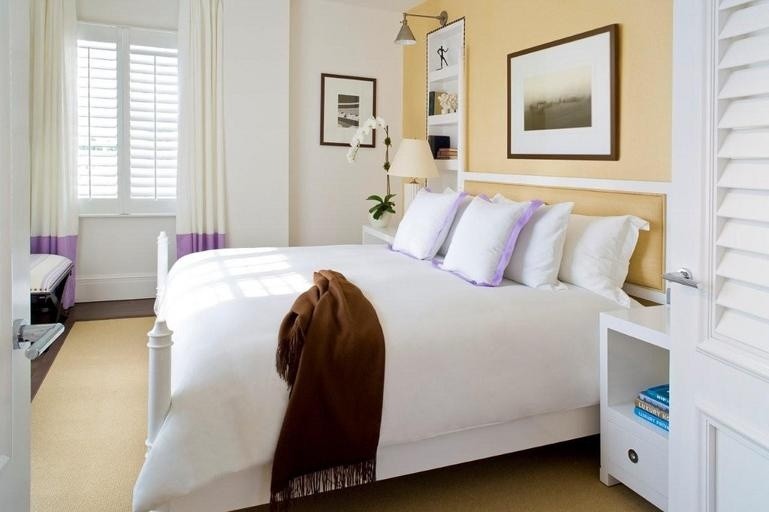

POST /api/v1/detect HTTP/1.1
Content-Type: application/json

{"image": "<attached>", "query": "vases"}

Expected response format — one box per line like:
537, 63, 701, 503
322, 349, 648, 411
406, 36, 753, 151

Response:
367, 210, 389, 229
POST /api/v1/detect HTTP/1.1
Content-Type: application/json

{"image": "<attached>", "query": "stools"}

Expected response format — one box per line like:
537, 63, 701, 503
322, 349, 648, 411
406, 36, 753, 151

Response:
30, 253, 75, 354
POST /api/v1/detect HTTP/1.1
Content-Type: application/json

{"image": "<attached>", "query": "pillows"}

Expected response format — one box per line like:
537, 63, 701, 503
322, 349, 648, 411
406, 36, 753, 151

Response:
387, 186, 651, 308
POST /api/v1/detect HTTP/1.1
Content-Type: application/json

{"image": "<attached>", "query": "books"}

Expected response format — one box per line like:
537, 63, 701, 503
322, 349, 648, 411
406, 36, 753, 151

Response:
646, 382, 670, 407
639, 389, 669, 414
633, 405, 669, 433
634, 396, 670, 423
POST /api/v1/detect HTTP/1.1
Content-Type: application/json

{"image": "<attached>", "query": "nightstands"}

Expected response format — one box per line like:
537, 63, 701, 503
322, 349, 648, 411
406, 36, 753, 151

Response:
362, 221, 399, 244
599, 304, 670, 512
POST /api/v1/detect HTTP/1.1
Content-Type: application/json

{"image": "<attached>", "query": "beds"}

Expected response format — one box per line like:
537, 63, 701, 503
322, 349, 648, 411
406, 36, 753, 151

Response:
130, 176, 665, 512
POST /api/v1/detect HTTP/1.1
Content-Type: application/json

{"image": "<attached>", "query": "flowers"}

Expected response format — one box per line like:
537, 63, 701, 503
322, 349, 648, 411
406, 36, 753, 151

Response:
345, 116, 396, 219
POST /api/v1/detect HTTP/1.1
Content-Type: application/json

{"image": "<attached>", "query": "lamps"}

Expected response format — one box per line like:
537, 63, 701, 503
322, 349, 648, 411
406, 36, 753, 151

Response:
393, 10, 448, 45
386, 138, 438, 216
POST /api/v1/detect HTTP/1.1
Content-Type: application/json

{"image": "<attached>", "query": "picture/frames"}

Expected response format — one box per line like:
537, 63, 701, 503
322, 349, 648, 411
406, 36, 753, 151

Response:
506, 24, 621, 162
320, 72, 376, 148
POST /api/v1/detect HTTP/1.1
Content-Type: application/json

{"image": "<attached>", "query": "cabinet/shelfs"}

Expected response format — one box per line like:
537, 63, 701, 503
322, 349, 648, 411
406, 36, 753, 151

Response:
424, 17, 465, 193
